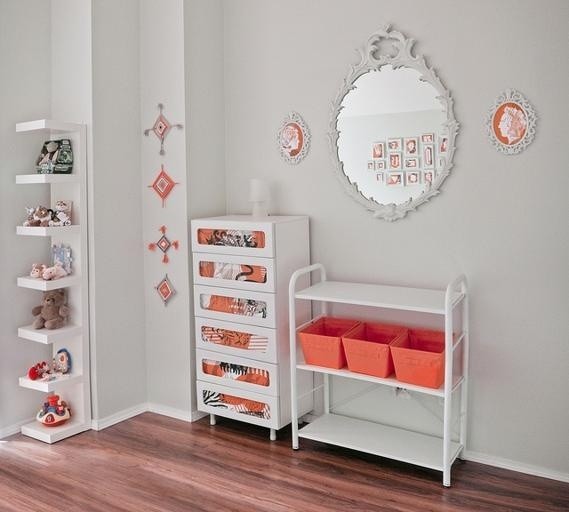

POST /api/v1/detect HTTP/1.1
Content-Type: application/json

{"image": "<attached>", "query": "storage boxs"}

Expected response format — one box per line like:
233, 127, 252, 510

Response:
299, 314, 461, 389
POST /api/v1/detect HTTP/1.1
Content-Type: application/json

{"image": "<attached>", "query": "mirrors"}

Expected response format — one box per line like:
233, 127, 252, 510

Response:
326, 21, 460, 224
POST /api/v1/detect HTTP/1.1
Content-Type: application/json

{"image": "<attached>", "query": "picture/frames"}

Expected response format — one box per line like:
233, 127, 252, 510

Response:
366, 131, 449, 189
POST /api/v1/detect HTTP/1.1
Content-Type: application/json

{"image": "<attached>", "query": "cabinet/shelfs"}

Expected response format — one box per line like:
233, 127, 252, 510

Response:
287, 262, 470, 489
191, 213, 315, 440
14, 119, 91, 445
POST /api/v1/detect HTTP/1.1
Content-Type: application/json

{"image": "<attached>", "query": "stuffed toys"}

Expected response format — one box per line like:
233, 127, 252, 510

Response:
22, 200, 72, 226
31, 288, 71, 329
30, 262, 68, 281
